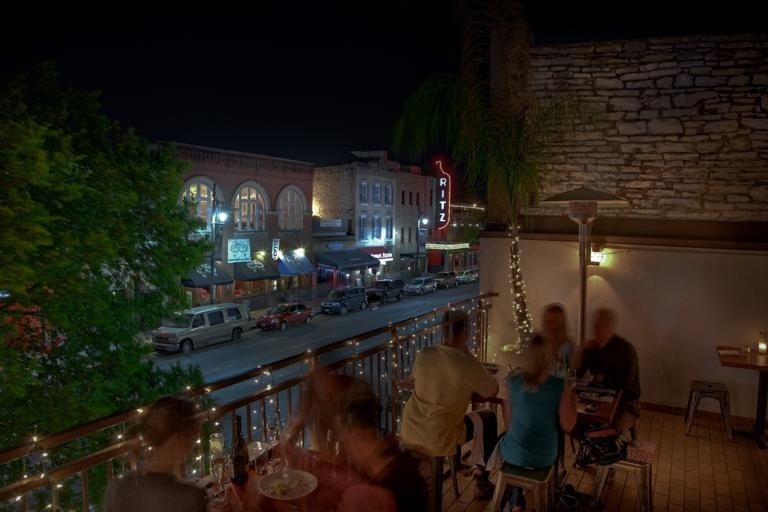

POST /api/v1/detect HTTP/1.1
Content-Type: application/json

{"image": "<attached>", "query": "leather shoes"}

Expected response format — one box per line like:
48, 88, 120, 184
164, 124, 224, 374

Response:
474, 481, 495, 500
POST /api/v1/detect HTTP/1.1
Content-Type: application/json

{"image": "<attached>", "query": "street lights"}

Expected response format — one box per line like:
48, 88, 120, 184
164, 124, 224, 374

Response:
209, 211, 228, 303
415, 216, 430, 274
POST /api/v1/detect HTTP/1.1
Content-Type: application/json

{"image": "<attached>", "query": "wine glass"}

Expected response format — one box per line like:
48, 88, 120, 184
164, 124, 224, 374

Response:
265, 423, 281, 464
326, 428, 342, 482
207, 431, 230, 508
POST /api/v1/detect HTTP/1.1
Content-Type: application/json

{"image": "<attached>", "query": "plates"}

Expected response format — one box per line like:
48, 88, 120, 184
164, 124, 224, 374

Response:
258, 469, 319, 500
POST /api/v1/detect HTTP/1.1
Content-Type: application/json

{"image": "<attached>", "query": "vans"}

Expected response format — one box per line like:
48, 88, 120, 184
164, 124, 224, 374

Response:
148, 301, 250, 357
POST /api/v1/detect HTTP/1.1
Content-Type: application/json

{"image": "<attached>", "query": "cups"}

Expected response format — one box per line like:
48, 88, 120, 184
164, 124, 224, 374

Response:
254, 446, 268, 473
740, 344, 750, 356
567, 367, 576, 392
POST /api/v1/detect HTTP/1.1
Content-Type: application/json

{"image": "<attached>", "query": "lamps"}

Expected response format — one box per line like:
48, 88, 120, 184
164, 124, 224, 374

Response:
589, 238, 606, 266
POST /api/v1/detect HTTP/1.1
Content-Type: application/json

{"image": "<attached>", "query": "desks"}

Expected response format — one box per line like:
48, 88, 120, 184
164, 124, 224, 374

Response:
716, 343, 767, 452
209, 447, 368, 512
396, 360, 625, 451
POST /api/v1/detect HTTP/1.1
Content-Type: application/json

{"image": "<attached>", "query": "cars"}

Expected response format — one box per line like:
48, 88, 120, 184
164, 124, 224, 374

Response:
405, 276, 440, 297
256, 301, 316, 333
457, 269, 478, 285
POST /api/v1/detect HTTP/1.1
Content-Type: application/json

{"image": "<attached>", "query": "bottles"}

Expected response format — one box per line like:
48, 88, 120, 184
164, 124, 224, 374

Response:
229, 416, 249, 482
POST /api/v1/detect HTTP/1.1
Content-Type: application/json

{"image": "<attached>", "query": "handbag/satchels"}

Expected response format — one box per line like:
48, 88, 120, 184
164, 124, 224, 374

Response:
577, 424, 625, 466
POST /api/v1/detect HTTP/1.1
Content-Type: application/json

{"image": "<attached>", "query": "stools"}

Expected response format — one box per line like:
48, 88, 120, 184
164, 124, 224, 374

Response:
415, 452, 459, 511
682, 379, 735, 442
487, 460, 556, 510
590, 458, 646, 509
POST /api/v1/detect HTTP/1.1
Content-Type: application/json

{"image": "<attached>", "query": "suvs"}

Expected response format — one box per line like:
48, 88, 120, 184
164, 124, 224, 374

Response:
433, 271, 459, 289
319, 286, 370, 315
366, 278, 406, 303
